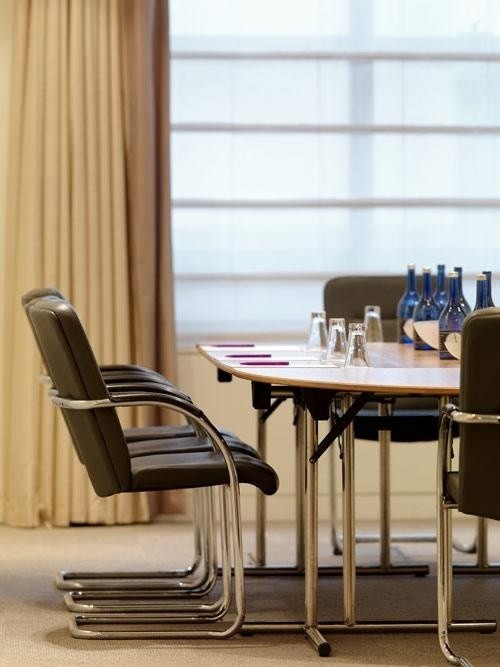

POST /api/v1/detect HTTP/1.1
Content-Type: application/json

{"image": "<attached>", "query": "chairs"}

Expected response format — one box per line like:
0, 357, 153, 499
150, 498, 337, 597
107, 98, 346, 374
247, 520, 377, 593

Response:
436, 307, 500, 667
323, 273, 480, 554
23, 289, 279, 639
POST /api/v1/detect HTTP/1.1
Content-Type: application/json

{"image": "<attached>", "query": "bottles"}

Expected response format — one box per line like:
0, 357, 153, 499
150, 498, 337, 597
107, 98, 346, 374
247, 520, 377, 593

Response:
432, 263, 495, 310
344, 322, 370, 368
325, 317, 347, 362
306, 311, 329, 357
364, 305, 384, 350
412, 267, 439, 351
396, 264, 421, 344
438, 272, 468, 360
